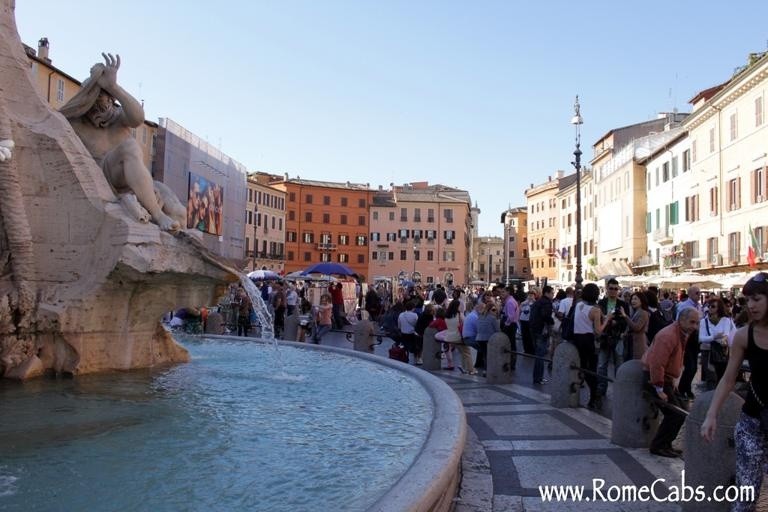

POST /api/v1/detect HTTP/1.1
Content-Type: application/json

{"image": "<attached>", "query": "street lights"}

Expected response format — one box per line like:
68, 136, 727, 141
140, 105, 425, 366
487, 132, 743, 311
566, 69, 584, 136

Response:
411, 244, 416, 282
573, 93, 585, 294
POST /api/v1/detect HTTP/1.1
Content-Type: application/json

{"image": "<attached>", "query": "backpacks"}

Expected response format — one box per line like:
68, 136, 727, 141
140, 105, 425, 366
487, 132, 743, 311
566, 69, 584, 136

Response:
646, 307, 668, 342
388, 343, 410, 364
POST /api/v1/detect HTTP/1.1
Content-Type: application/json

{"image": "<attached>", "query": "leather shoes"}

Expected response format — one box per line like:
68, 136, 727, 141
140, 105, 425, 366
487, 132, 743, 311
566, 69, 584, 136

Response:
649, 442, 684, 458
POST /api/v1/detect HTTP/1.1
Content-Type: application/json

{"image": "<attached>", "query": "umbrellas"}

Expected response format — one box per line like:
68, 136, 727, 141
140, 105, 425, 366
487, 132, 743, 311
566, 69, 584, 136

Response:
246, 262, 355, 284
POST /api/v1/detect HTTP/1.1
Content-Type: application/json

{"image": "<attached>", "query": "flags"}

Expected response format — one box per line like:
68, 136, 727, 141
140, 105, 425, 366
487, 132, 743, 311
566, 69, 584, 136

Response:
747, 225, 760, 270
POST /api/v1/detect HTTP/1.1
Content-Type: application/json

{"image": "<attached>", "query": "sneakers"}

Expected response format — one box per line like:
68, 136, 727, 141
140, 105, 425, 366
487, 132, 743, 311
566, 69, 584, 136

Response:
414, 358, 424, 365
458, 364, 487, 378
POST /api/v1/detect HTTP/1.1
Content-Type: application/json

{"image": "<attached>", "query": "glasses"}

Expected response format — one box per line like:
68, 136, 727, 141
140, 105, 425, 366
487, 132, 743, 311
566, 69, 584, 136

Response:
607, 286, 619, 291
707, 304, 718, 309
752, 273, 768, 284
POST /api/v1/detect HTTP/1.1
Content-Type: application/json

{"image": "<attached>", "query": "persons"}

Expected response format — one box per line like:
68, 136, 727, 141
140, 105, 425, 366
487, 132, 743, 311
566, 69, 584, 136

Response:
168, 273, 768, 511
65, 52, 181, 234
187, 179, 223, 236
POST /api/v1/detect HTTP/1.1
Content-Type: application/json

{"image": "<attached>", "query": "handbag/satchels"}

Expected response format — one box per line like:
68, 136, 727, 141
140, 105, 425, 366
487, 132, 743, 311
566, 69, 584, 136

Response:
707, 339, 730, 368
558, 303, 577, 342
